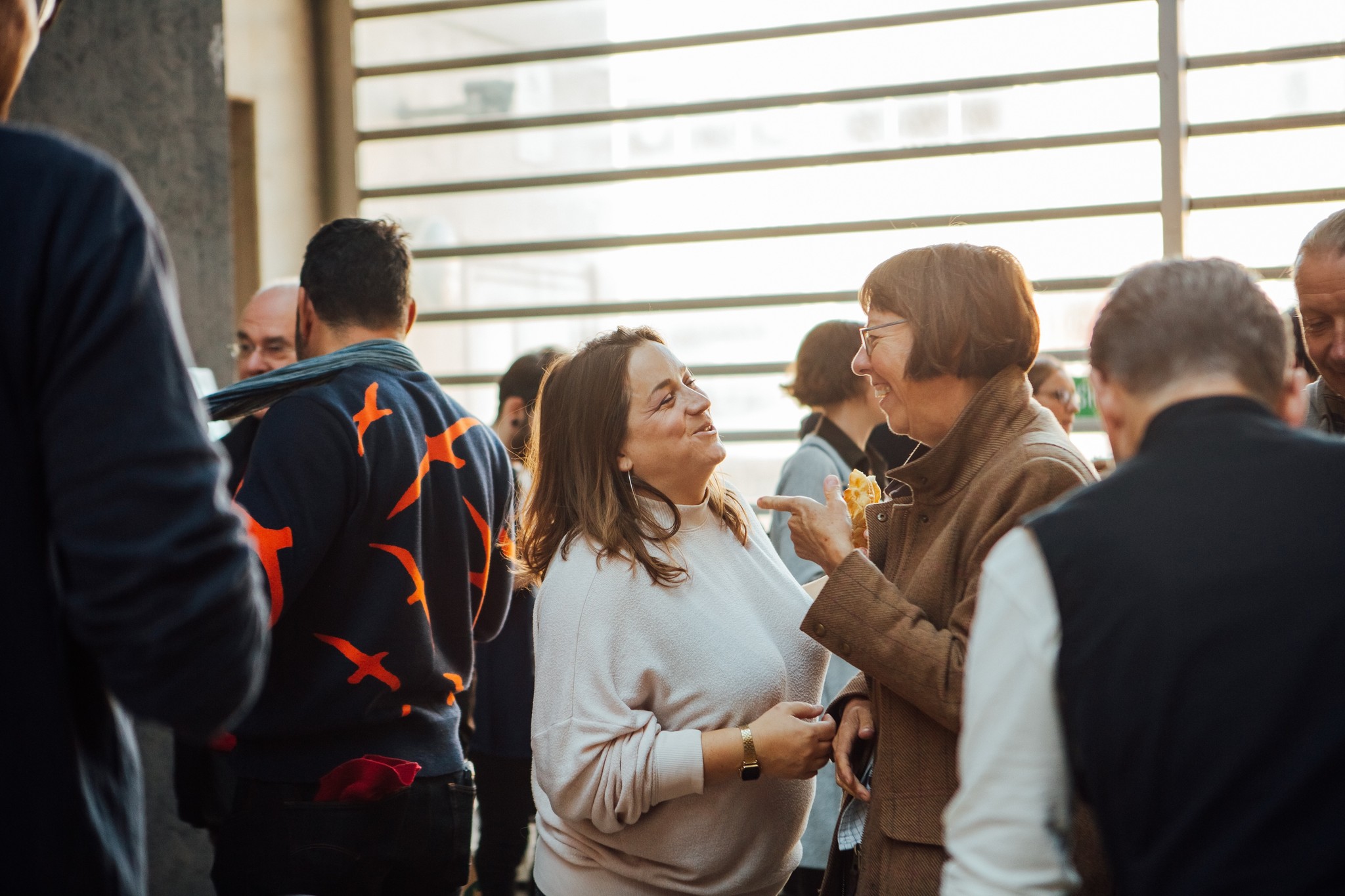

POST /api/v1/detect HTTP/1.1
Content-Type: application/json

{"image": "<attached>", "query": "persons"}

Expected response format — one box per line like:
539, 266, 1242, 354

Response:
166, 216, 569, 896
522, 327, 833, 896
758, 200, 1344, 896
0, 0, 269, 896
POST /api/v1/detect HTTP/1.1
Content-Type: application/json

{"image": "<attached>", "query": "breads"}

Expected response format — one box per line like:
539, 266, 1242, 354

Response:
842, 468, 881, 550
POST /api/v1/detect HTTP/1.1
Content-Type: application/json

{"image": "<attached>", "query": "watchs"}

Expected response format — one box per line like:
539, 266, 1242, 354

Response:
736, 724, 762, 781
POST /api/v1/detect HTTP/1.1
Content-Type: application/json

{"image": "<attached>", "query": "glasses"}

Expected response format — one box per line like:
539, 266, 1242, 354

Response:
227, 341, 292, 362
858, 319, 909, 355
1042, 386, 1080, 406
